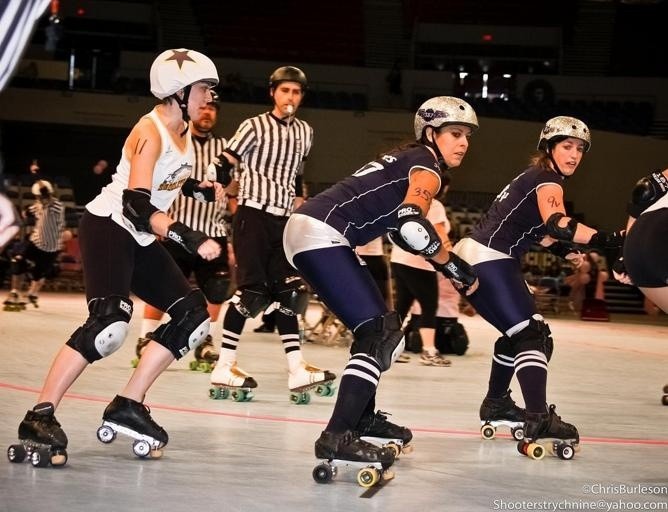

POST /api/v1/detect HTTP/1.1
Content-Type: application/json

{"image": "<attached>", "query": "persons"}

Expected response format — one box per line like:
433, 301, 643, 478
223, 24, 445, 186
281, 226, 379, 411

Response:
227, 170, 470, 367
6, 48, 229, 470
132, 87, 230, 372
610, 163, 668, 406
449, 115, 606, 460
281, 96, 481, 488
0, 177, 82, 313
204, 64, 338, 402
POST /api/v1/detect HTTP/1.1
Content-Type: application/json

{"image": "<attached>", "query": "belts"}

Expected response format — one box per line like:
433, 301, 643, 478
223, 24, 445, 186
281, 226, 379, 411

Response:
236, 199, 290, 217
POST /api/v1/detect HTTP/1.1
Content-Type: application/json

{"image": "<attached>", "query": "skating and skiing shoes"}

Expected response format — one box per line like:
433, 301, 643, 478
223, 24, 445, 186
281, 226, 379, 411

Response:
479, 390, 527, 440
359, 409, 413, 459
97, 394, 169, 457
189, 334, 220, 372
313, 430, 395, 486
517, 403, 581, 460
7, 401, 67, 467
208, 362, 258, 402
21, 292, 39, 309
286, 362, 336, 404
130, 333, 153, 367
3, 290, 21, 312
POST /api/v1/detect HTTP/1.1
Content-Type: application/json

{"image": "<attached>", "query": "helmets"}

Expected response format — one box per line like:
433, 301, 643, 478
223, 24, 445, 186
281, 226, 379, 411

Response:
31, 180, 54, 196
149, 49, 219, 101
414, 96, 479, 142
269, 66, 308, 87
207, 90, 221, 109
537, 116, 591, 155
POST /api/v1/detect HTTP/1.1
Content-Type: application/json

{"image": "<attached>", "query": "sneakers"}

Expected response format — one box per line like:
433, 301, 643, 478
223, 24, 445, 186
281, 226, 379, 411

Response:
420, 349, 451, 367
394, 354, 410, 362
253, 323, 275, 332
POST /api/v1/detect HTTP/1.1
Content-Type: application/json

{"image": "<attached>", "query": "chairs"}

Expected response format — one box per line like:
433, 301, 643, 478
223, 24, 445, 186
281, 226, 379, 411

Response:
520, 246, 583, 316
5, 182, 84, 292
444, 203, 486, 244
